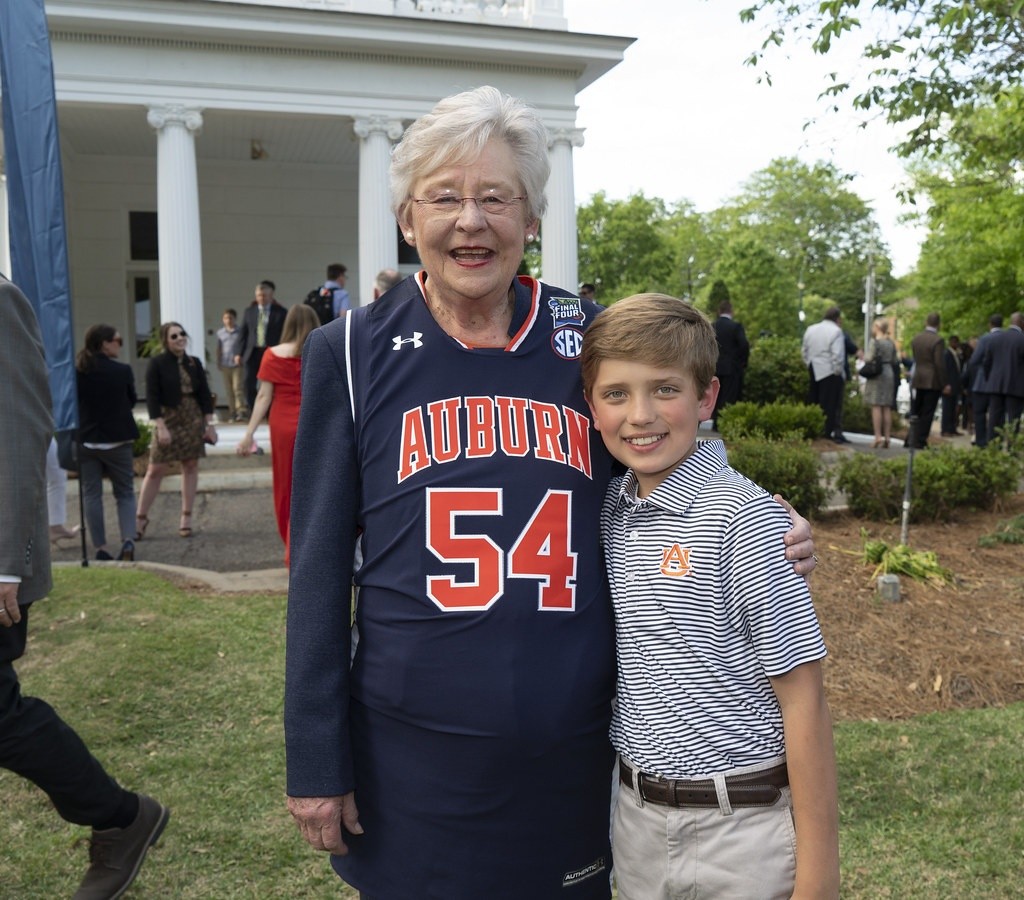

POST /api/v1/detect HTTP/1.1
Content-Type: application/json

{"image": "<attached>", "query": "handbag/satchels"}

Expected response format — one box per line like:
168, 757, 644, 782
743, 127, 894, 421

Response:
895, 375, 913, 416
858, 338, 883, 378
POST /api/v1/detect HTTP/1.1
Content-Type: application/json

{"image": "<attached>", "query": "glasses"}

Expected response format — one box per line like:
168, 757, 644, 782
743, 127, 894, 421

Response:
407, 191, 528, 215
166, 331, 186, 339
110, 338, 123, 346
578, 291, 589, 295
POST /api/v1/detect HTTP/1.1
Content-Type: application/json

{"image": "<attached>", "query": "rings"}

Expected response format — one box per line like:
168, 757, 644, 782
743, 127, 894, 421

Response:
811, 556, 820, 565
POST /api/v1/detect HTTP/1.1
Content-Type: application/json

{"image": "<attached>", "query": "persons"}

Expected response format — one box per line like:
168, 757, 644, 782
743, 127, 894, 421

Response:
239, 305, 324, 569
45, 437, 82, 552
577, 292, 840, 900
800, 307, 900, 449
0, 269, 169, 900
67, 322, 137, 562
707, 302, 751, 432
581, 285, 606, 311
216, 264, 400, 421
138, 322, 216, 540
905, 312, 1024, 450
280, 88, 819, 900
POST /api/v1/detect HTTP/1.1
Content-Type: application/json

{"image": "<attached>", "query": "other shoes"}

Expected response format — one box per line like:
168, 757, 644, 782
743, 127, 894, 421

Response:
235, 411, 249, 421
227, 412, 236, 423
834, 434, 851, 444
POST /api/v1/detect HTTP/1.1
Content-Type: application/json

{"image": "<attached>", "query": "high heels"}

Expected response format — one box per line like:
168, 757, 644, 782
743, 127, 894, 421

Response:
95, 549, 115, 561
868, 439, 883, 449
133, 513, 150, 541
178, 511, 193, 537
884, 439, 891, 448
115, 541, 135, 561
49, 524, 82, 551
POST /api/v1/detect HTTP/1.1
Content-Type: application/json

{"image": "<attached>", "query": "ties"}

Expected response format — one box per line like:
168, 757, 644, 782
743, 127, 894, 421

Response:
256, 309, 266, 347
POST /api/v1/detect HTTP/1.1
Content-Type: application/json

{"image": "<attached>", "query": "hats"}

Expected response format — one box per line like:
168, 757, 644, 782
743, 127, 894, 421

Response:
872, 318, 890, 333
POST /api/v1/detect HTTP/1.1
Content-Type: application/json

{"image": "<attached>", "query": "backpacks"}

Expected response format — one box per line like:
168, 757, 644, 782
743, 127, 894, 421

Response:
302, 286, 340, 326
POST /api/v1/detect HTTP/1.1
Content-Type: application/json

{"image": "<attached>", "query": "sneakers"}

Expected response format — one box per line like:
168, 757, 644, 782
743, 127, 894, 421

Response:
68, 792, 170, 900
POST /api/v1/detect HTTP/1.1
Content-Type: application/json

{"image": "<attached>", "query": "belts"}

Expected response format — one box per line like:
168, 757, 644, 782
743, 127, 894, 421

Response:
618, 758, 790, 808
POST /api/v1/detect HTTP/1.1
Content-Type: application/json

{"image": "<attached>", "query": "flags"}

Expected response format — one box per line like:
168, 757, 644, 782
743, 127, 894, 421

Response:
0, 0, 81, 434
